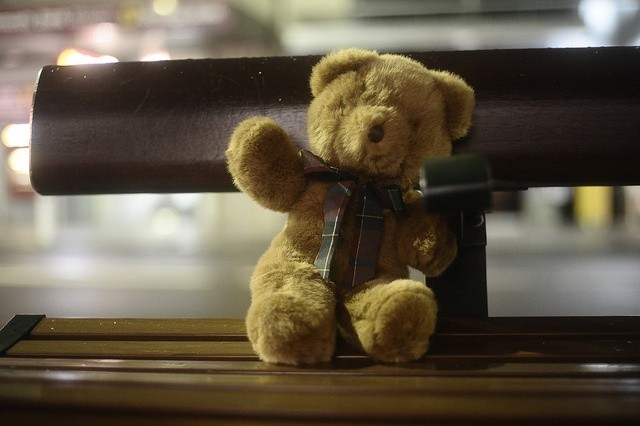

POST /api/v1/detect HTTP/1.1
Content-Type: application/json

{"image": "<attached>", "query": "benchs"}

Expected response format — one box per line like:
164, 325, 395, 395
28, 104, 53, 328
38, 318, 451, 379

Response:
1, 45, 640, 426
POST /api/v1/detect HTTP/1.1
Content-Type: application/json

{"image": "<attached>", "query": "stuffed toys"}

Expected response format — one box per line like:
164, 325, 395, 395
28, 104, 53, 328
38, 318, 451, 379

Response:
225, 48, 478, 368
